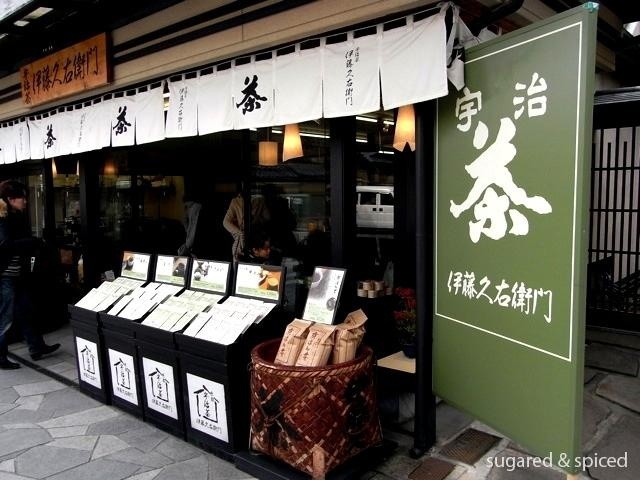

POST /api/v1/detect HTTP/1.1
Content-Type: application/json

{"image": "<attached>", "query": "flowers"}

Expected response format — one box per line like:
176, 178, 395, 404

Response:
393, 287, 417, 342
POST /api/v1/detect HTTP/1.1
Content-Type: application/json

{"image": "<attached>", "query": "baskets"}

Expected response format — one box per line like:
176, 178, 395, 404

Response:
249, 338, 385, 480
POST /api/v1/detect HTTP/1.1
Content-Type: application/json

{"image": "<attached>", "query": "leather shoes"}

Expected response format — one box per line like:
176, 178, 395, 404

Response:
30, 343, 60, 360
0, 360, 20, 369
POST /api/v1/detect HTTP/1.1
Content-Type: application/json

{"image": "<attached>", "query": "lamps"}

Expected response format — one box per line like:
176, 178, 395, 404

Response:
281, 124, 305, 163
52, 156, 118, 180
390, 105, 417, 152
257, 127, 278, 169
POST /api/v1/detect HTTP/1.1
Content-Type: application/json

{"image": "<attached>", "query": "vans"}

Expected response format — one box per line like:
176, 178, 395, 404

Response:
355, 184, 394, 229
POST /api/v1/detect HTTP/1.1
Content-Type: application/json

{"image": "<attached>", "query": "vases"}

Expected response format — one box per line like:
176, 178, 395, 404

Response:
402, 342, 416, 359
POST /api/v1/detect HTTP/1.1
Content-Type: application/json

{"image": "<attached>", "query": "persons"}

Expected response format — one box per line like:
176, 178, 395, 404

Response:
178, 184, 332, 276
0, 179, 60, 369
